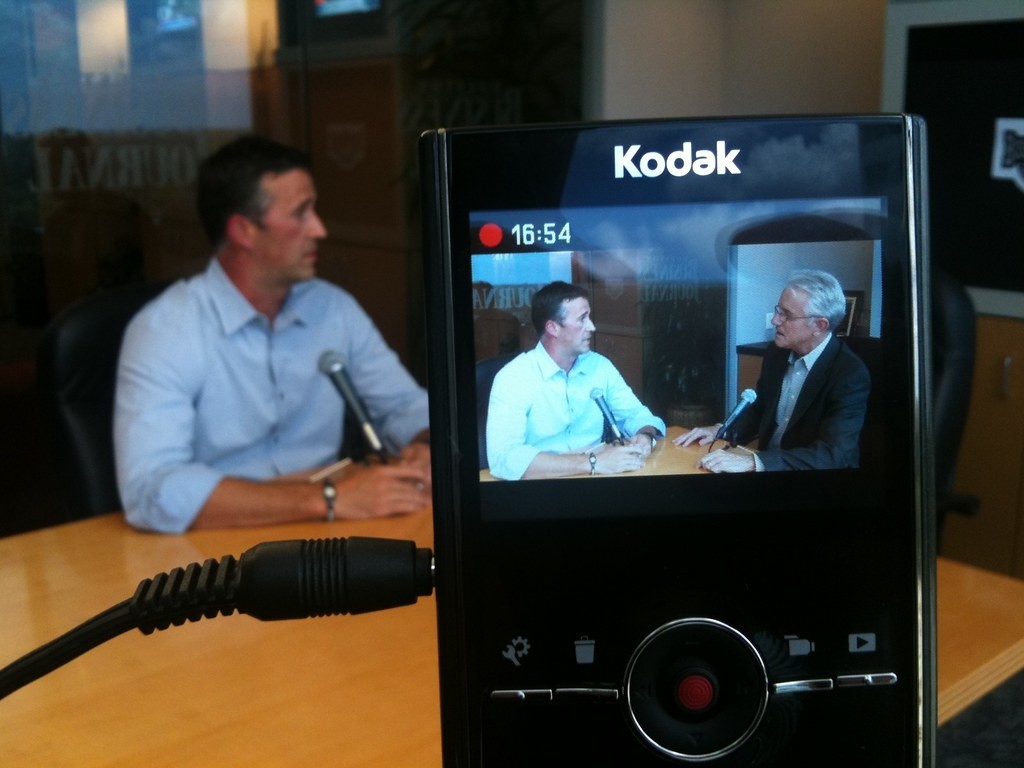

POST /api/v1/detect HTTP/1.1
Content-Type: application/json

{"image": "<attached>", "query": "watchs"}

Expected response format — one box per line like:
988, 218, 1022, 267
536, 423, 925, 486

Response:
643, 431, 657, 453
590, 452, 597, 475
323, 480, 337, 522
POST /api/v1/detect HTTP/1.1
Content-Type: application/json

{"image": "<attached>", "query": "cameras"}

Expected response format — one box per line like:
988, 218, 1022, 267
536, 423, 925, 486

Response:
416, 112, 935, 766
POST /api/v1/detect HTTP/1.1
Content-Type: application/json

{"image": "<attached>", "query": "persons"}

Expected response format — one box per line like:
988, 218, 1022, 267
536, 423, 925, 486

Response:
672, 267, 872, 473
486, 281, 668, 480
108, 136, 436, 536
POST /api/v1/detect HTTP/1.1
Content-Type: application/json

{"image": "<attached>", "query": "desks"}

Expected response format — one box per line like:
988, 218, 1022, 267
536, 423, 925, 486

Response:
0, 454, 1024, 767
479, 427, 754, 484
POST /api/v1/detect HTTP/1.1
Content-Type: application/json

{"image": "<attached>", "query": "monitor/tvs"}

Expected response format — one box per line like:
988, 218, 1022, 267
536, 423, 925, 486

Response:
879, 0, 1024, 322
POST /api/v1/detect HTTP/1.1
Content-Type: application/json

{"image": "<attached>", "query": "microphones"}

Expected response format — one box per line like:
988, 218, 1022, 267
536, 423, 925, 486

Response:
714, 388, 757, 440
590, 387, 624, 446
316, 351, 388, 467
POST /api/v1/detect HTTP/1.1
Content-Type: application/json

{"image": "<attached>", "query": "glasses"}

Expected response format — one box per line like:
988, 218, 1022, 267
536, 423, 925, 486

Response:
775, 304, 822, 322
